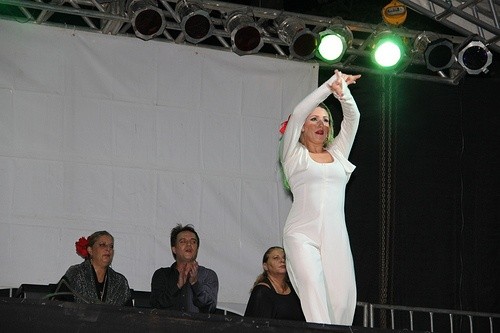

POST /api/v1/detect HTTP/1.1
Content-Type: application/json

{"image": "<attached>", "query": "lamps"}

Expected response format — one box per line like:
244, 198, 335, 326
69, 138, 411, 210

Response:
125, 0, 493, 74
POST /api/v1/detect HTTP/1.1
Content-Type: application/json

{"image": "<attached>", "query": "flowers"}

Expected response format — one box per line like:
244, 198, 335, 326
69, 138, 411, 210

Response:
75, 236, 88, 262
280, 115, 293, 134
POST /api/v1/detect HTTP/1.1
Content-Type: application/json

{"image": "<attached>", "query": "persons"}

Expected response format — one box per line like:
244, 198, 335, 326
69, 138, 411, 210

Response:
151, 224, 218, 314
279, 69, 361, 326
49, 231, 133, 307
243, 247, 306, 321
